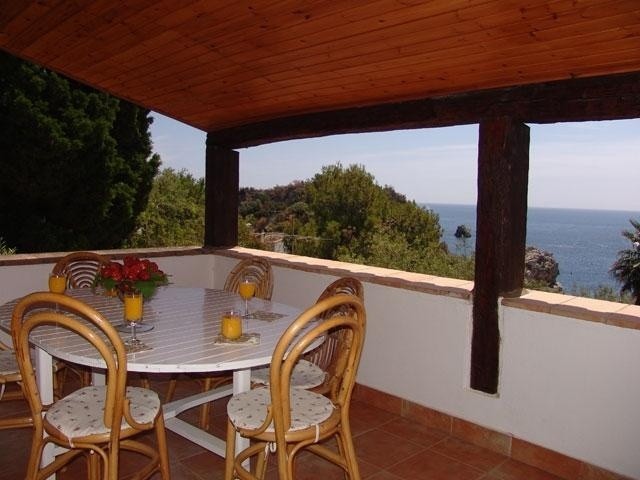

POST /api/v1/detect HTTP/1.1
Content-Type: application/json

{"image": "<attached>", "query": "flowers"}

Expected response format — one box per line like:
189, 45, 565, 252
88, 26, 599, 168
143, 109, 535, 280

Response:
91, 255, 174, 304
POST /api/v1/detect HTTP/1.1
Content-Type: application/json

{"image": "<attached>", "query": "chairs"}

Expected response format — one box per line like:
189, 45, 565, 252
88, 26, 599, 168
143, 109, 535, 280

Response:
10, 291, 171, 480
250, 276, 364, 398
0, 325, 66, 430
52, 251, 151, 398
164, 255, 274, 431
224, 294, 367, 480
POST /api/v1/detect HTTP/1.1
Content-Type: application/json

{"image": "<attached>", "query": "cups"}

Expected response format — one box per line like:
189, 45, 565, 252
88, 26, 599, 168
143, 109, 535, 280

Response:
48, 272, 67, 315
123, 289, 143, 345
239, 277, 257, 318
221, 311, 243, 340
